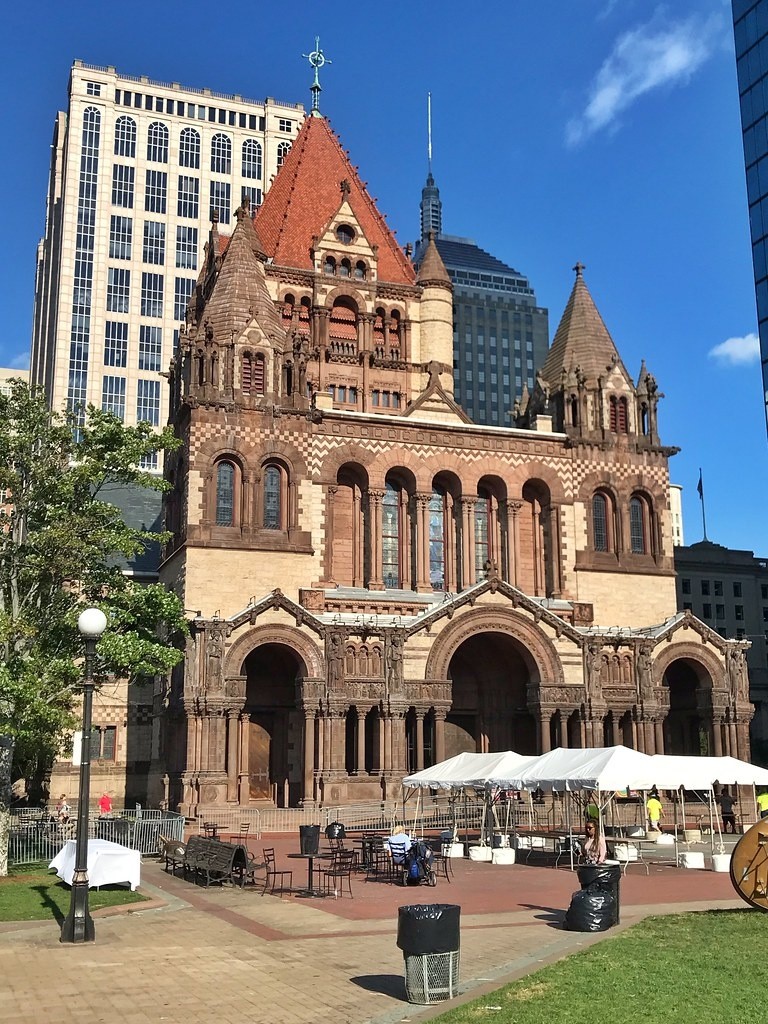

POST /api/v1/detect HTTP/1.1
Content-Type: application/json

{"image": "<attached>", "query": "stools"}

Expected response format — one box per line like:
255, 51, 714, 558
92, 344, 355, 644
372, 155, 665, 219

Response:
443, 823, 753, 873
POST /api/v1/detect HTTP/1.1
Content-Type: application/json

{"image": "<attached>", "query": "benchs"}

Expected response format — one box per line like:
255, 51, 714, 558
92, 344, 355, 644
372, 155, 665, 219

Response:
164, 835, 268, 889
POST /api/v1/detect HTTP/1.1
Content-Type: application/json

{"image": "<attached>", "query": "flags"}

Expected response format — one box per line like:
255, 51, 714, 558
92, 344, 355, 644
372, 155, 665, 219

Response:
696, 478, 702, 498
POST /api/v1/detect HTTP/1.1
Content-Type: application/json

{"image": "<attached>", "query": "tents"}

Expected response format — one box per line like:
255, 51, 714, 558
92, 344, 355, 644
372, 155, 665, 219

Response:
392, 745, 768, 871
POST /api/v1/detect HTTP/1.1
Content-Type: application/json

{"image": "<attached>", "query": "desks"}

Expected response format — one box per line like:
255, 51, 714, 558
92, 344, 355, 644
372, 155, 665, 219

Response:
577, 836, 654, 876
48, 838, 141, 891
200, 826, 228, 838
288, 853, 336, 898
519, 832, 566, 868
353, 837, 387, 870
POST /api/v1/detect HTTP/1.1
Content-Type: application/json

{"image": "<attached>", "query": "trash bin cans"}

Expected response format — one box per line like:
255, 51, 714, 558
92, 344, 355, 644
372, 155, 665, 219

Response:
398, 904, 461, 1005
577, 863, 621, 927
299, 825, 320, 855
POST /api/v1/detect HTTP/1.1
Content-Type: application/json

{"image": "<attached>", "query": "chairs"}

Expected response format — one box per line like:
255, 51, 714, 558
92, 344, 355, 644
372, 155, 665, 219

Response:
199, 822, 455, 899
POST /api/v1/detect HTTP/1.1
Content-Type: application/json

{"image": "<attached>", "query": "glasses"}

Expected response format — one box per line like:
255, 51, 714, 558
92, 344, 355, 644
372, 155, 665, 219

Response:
586, 826, 593, 830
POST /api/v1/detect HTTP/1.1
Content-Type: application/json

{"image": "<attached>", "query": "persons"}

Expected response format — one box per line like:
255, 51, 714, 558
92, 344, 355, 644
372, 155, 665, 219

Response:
97, 792, 113, 814
586, 644, 602, 692
388, 636, 403, 692
637, 646, 653, 694
717, 788, 736, 833
729, 650, 745, 694
386, 825, 412, 865
647, 792, 665, 833
327, 634, 346, 687
581, 819, 606, 865
204, 631, 225, 691
56, 794, 66, 810
756, 786, 768, 819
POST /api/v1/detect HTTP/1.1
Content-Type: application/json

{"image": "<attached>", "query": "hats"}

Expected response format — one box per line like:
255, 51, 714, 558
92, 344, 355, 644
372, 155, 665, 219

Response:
649, 792, 656, 797
